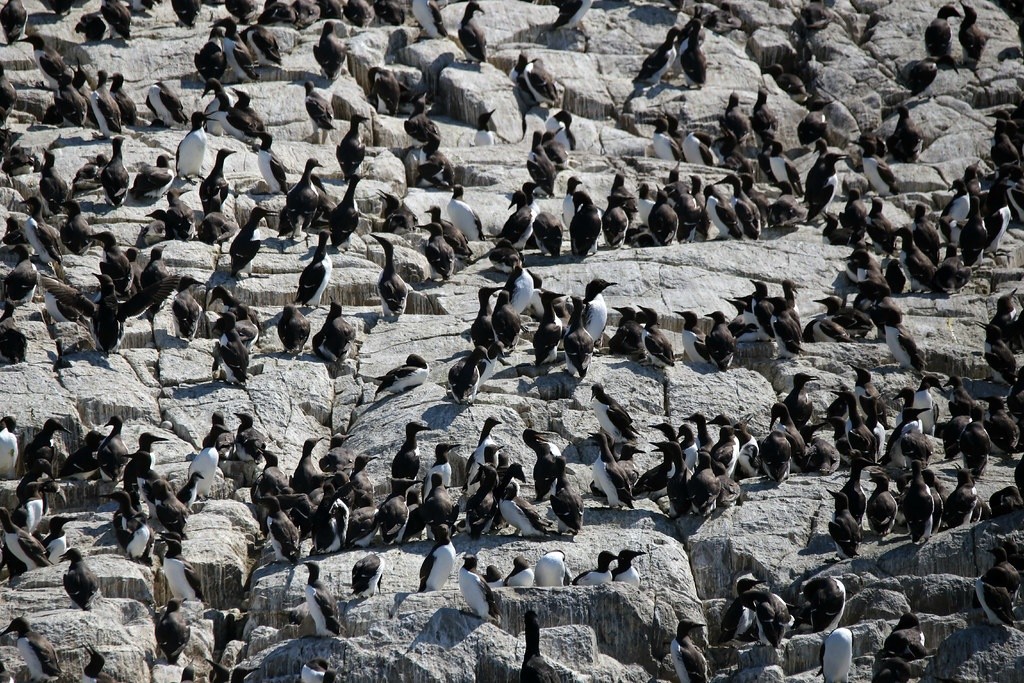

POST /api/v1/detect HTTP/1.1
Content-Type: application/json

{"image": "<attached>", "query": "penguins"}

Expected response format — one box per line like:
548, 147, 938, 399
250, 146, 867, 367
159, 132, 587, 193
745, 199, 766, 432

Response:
0, 0, 1022, 683
